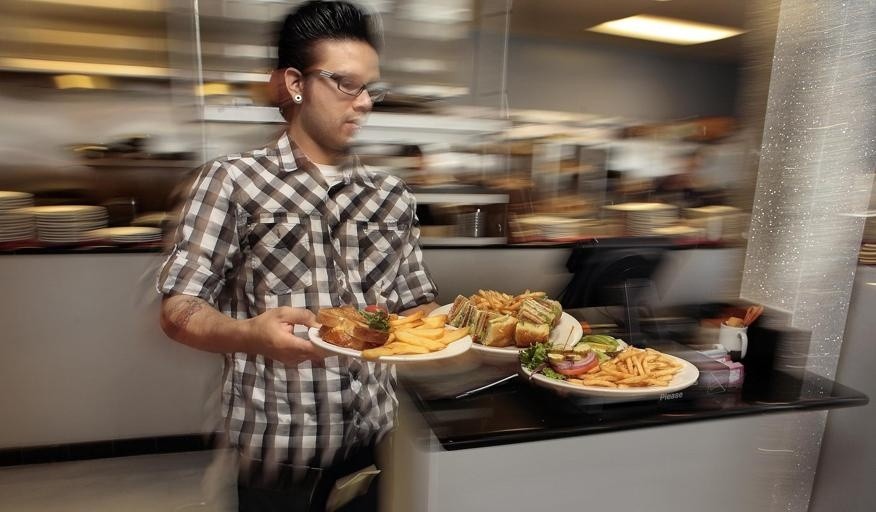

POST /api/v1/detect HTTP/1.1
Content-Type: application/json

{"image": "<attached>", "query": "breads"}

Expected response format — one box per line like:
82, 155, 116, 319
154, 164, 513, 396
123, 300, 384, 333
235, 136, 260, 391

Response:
314, 305, 389, 350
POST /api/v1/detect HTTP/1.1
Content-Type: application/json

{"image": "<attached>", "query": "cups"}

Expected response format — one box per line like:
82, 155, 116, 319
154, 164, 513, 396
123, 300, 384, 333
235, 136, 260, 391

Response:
717, 321, 749, 359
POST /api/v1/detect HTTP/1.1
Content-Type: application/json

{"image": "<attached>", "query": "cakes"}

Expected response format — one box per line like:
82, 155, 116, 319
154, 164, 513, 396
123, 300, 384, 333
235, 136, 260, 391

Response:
446, 289, 564, 346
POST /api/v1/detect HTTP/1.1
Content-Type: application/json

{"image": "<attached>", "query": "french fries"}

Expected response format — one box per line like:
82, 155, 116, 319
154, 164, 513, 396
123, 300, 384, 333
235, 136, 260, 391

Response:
363, 309, 471, 360
568, 346, 683, 390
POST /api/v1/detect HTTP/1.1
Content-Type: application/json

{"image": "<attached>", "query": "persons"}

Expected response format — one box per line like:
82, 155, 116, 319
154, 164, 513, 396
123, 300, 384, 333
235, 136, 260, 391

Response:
154, 0, 444, 510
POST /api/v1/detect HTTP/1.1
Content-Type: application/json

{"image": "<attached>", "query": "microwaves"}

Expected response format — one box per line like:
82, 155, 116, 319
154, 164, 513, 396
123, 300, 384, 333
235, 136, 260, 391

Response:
409, 187, 512, 247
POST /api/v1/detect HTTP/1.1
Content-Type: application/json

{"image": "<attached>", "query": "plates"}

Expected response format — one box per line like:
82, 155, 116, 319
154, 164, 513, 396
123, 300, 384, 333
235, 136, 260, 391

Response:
426, 301, 584, 354
0, 187, 181, 245
509, 200, 747, 245
308, 316, 473, 361
518, 345, 699, 400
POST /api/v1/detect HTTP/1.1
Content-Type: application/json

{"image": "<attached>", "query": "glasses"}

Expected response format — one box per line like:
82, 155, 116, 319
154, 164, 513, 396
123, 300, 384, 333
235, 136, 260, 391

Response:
304, 66, 390, 103
